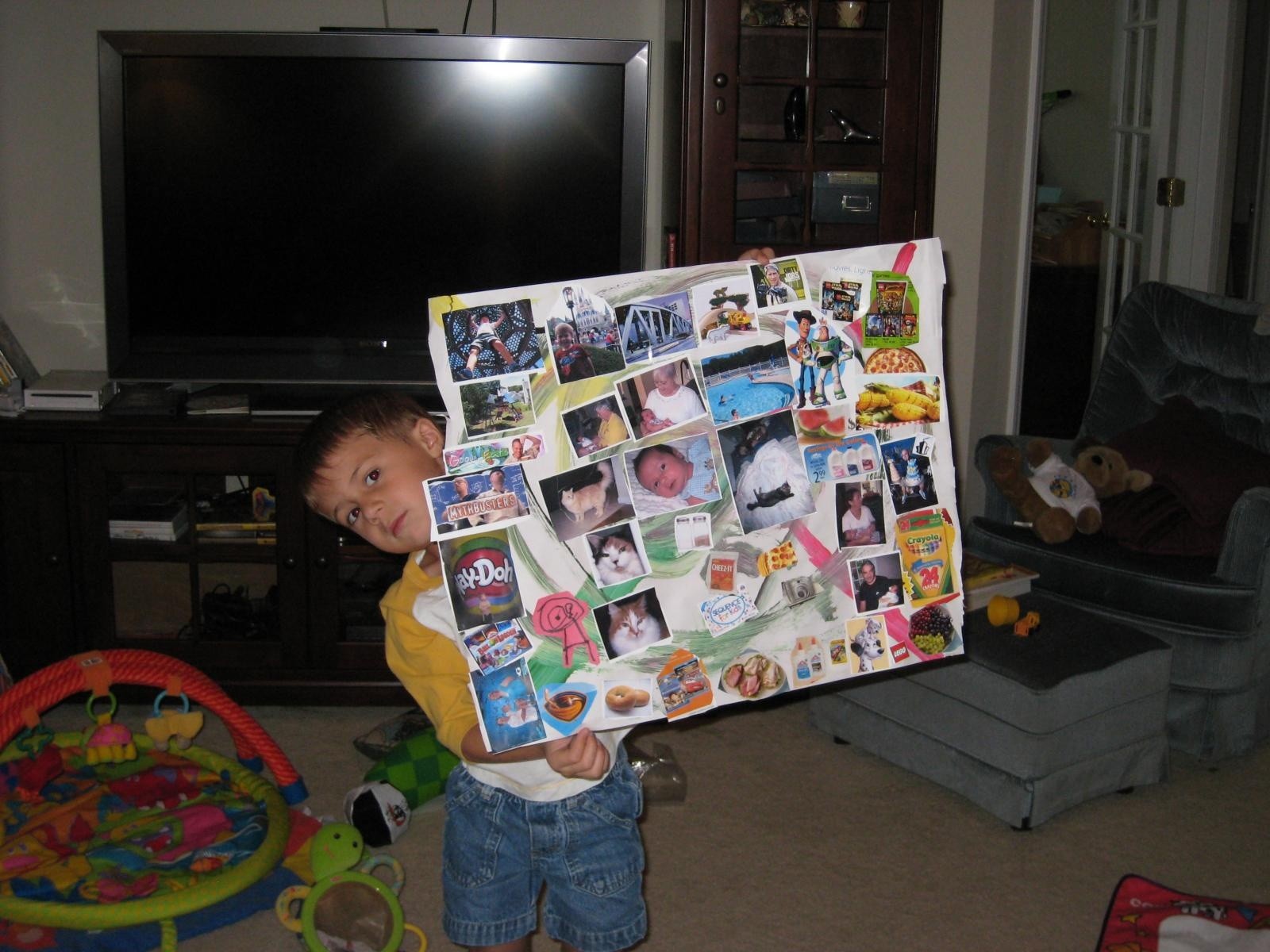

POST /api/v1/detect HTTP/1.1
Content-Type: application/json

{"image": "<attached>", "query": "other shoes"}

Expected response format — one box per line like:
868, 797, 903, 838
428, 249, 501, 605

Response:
456, 369, 473, 380
901, 495, 905, 504
919, 490, 927, 500
509, 364, 521, 372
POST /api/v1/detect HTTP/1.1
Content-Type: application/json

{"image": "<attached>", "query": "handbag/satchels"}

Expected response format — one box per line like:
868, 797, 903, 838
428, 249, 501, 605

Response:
201, 583, 268, 640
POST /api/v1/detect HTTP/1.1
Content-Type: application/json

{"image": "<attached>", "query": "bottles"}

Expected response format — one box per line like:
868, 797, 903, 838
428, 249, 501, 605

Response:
808, 637, 824, 677
794, 643, 812, 681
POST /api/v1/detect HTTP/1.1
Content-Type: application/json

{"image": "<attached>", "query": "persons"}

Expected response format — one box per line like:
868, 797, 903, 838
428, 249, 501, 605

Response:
432, 258, 950, 728
299, 390, 649, 952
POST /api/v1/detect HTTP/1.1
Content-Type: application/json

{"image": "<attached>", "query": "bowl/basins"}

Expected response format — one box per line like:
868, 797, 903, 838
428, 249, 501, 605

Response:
909, 605, 955, 654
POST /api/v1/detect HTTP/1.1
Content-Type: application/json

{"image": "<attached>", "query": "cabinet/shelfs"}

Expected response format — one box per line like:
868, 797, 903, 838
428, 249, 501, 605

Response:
1, 383, 445, 706
679, 0, 942, 266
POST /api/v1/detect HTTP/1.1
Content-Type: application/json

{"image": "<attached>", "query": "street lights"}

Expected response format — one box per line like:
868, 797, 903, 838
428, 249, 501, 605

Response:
562, 286, 581, 344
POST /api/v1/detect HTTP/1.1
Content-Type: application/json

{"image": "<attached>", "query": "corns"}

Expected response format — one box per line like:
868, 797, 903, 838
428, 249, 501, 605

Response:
858, 389, 940, 421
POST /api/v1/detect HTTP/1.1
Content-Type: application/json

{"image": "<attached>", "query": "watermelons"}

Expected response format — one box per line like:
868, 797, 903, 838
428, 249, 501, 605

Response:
796, 409, 847, 438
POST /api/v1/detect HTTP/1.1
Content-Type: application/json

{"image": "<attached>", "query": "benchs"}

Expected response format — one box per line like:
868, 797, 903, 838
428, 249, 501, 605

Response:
810, 594, 1174, 832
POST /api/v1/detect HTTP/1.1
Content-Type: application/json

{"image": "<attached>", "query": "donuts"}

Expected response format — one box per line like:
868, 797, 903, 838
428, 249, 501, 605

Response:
606, 685, 650, 712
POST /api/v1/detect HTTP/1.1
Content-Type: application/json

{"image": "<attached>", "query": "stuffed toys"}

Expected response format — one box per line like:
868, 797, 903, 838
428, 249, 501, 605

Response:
972, 434, 1154, 547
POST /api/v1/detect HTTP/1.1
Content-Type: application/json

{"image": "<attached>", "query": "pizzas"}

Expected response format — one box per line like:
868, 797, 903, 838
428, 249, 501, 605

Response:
865, 348, 924, 372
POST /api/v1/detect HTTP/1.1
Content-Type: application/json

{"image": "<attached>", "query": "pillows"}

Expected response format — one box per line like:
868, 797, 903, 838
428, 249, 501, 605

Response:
1091, 394, 1270, 556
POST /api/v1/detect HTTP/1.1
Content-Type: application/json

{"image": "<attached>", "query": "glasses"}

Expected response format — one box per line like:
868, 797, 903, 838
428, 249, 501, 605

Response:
454, 480, 466, 484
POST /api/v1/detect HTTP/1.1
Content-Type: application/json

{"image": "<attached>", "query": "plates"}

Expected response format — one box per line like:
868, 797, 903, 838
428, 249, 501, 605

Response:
721, 652, 786, 701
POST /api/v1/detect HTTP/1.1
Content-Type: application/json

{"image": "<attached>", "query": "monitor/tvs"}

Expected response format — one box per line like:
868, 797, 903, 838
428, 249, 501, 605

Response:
96, 25, 650, 384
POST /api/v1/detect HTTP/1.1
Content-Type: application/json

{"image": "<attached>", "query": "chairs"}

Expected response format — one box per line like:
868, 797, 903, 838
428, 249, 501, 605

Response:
964, 280, 1270, 770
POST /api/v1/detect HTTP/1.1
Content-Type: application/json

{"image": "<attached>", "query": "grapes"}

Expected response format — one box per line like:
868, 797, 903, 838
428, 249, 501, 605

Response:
909, 605, 953, 654
